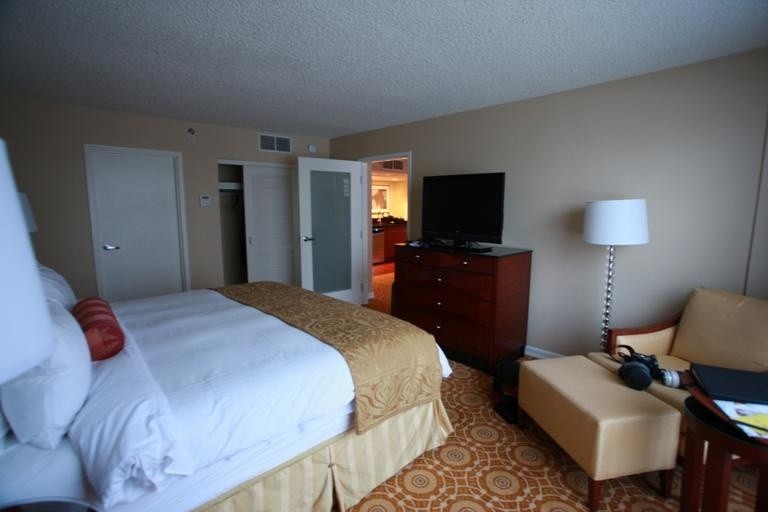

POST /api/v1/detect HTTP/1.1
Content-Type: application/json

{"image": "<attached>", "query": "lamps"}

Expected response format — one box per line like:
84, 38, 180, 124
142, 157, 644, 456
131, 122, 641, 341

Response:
583, 198, 648, 354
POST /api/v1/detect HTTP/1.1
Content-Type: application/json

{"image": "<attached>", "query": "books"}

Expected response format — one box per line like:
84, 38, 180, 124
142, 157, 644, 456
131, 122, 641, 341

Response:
690, 363, 768, 403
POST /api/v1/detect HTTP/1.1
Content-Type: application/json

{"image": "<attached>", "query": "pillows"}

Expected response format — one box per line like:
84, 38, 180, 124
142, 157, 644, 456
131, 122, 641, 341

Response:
69, 295, 126, 362
36, 264, 80, 314
2, 295, 91, 449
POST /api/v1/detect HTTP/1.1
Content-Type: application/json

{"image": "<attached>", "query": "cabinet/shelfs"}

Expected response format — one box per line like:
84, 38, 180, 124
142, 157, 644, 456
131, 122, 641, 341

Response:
218, 160, 300, 284
391, 247, 533, 370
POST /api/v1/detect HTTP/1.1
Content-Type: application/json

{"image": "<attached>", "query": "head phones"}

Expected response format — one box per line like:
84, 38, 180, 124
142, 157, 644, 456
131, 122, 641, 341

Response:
611, 344, 659, 390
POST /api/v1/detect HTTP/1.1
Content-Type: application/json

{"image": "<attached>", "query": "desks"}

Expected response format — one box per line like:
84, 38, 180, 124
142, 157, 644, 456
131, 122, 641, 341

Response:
679, 397, 767, 512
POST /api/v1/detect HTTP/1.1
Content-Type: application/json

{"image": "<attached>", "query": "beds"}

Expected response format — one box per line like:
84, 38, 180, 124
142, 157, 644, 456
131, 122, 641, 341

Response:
82, 280, 449, 512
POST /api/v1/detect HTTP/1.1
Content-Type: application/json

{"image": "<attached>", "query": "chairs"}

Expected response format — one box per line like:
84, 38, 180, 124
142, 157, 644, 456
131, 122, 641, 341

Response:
606, 286, 766, 500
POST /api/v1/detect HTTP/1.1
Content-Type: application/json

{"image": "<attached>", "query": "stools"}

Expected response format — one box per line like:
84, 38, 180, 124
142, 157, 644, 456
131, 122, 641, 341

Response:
519, 352, 681, 511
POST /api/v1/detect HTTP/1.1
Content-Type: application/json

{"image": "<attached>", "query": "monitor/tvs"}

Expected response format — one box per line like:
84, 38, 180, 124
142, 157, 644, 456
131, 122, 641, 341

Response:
421, 171, 506, 252
